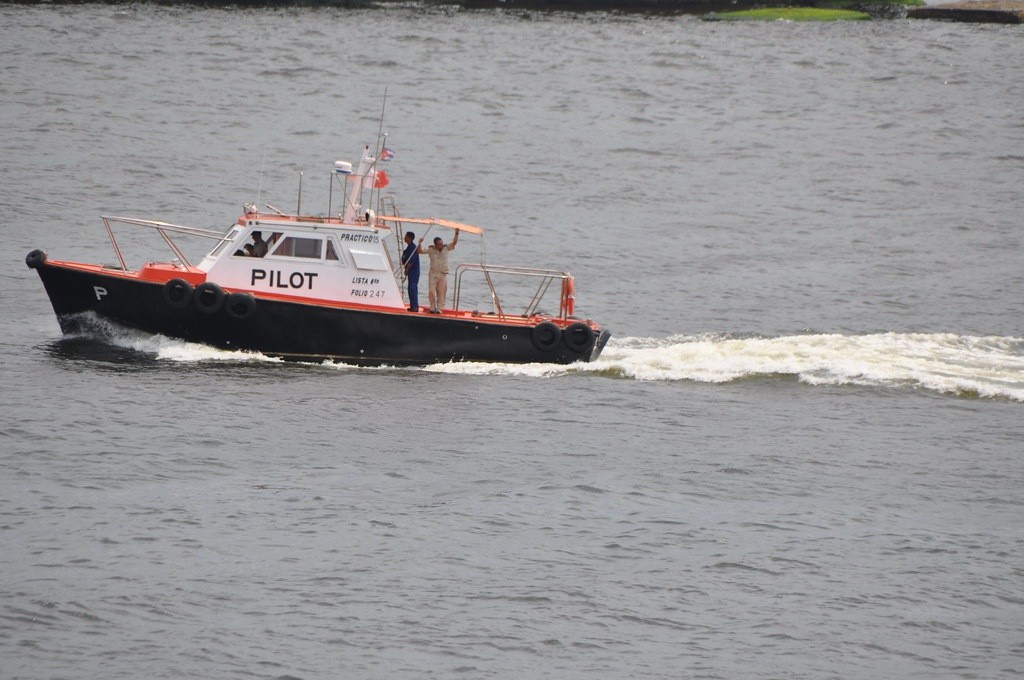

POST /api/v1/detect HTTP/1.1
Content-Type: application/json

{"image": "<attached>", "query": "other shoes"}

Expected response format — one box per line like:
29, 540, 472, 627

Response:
407, 308, 418, 312
430, 309, 441, 314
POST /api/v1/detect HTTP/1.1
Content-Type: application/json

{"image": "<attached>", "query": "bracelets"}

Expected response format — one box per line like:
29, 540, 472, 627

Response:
455, 233, 458, 235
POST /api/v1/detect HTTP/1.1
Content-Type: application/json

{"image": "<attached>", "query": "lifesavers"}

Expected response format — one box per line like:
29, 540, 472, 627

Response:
163, 278, 195, 311
562, 293, 574, 315
26, 250, 46, 268
563, 322, 595, 354
531, 321, 563, 353
561, 275, 574, 295
225, 292, 257, 321
193, 281, 226, 314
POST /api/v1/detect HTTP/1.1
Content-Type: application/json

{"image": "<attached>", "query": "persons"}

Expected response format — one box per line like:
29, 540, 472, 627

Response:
417, 228, 460, 314
401, 232, 420, 312
244, 231, 268, 258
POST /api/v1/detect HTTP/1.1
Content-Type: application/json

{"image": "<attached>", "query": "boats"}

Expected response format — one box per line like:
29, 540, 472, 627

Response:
25, 83, 613, 368
